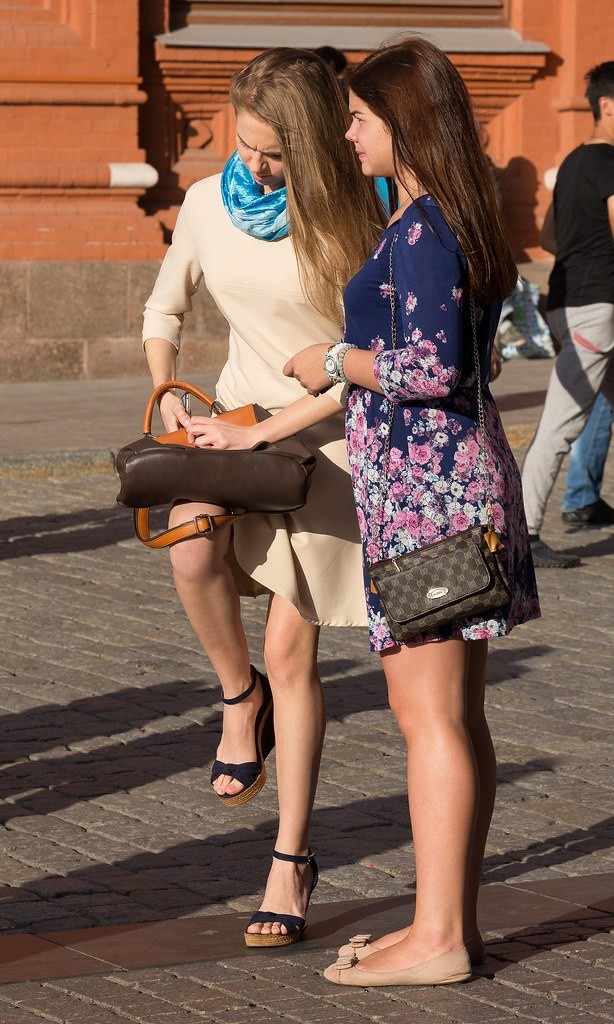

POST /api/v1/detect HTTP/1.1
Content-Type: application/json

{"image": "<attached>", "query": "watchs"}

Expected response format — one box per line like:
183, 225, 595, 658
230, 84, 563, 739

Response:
324, 342, 350, 381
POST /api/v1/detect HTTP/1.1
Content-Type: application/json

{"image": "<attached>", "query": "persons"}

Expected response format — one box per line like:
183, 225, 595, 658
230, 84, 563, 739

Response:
141, 46, 390, 955
282, 35, 542, 989
513, 60, 614, 570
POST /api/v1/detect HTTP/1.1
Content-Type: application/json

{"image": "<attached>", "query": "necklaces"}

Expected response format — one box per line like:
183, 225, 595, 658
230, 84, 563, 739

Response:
400, 186, 421, 208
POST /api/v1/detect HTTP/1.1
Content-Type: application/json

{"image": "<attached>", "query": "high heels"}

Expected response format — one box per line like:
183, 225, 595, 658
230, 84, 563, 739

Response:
210, 664, 276, 807
242, 849, 319, 948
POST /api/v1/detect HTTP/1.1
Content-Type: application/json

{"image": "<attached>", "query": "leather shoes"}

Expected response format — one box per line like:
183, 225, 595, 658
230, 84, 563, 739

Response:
338, 930, 487, 964
323, 947, 472, 987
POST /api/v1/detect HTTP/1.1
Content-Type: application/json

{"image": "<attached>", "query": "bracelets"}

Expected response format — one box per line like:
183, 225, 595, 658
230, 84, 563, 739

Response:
339, 344, 358, 385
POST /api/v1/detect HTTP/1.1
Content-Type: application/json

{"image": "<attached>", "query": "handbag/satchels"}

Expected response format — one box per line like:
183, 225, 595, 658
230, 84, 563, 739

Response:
368, 525, 514, 643
115, 380, 316, 550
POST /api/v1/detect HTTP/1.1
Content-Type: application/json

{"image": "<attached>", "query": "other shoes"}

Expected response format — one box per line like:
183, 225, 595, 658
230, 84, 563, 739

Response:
561, 498, 614, 529
530, 534, 580, 568
